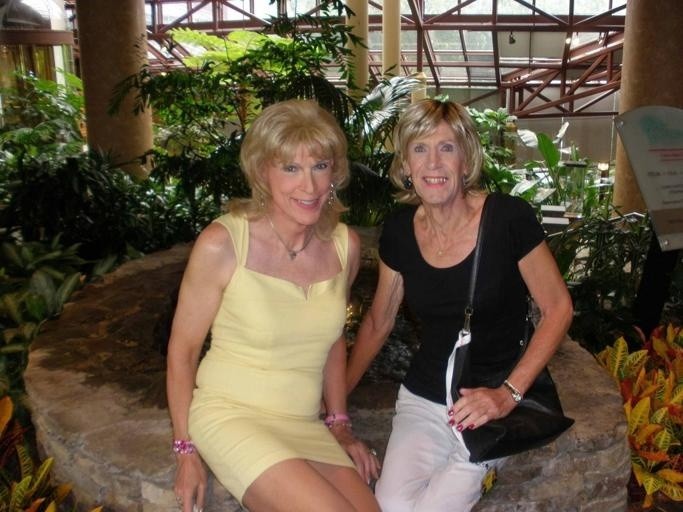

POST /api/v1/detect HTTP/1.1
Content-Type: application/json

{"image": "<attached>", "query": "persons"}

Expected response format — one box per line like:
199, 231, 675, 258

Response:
165, 95, 383, 511
318, 97, 574, 512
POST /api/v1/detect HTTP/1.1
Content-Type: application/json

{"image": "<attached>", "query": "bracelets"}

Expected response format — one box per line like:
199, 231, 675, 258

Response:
324, 413, 351, 429
503, 379, 524, 407
171, 438, 195, 455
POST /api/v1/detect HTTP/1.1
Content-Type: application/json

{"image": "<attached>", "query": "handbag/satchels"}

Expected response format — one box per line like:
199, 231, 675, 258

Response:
445, 329, 575, 464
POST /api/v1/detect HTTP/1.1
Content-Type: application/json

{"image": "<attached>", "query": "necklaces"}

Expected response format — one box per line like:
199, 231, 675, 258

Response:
423, 195, 476, 256
262, 207, 315, 262
